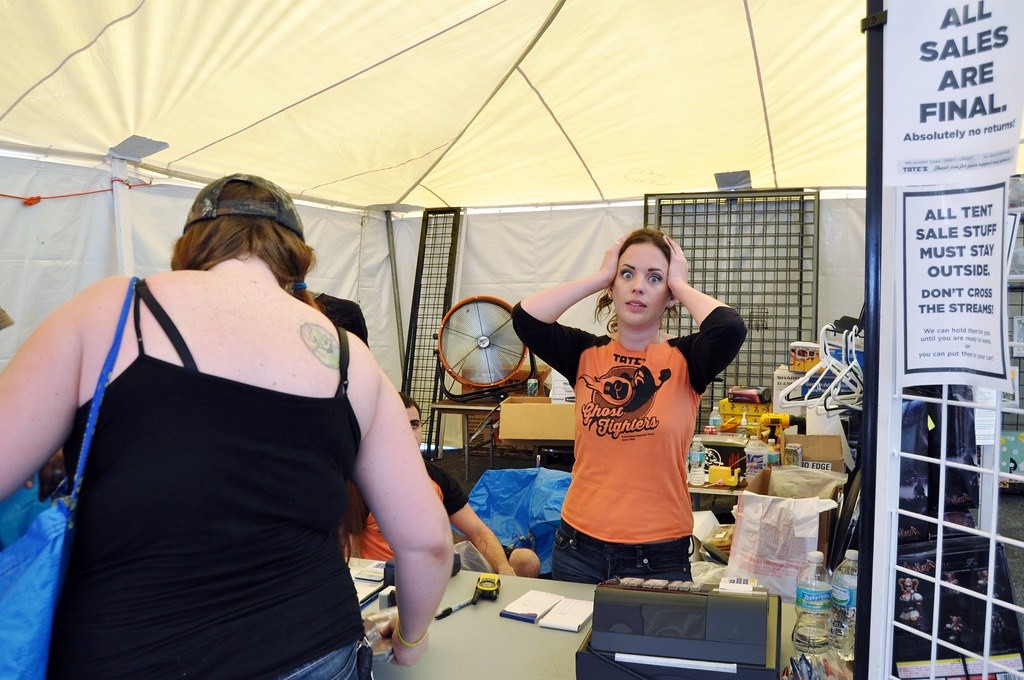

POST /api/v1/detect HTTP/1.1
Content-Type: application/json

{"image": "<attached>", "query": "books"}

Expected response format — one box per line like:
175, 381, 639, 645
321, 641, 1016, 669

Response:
499, 590, 595, 631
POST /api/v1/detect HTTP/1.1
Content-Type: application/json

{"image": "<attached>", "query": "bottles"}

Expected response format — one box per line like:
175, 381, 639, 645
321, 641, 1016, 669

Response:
829, 550, 858, 662
792, 550, 832, 659
689, 438, 705, 485
709, 406, 721, 433
743, 436, 780, 484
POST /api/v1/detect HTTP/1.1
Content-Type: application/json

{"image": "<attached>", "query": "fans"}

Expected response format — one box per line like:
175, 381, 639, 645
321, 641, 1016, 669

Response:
432, 295, 538, 403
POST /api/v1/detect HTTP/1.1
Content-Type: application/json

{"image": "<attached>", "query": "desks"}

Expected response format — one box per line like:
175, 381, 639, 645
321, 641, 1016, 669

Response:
343, 403, 855, 680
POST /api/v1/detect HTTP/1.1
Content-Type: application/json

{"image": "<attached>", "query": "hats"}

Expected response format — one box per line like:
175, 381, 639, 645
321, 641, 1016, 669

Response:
183, 173, 305, 242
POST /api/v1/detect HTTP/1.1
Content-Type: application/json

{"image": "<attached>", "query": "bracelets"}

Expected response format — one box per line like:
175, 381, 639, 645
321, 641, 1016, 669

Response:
396, 614, 429, 647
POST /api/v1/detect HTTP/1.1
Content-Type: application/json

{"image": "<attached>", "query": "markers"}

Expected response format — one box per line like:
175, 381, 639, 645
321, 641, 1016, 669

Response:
434, 597, 474, 621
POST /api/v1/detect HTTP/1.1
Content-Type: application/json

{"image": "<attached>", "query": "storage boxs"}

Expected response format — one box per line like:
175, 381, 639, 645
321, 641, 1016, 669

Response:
719, 423, 758, 436
778, 424, 846, 498
721, 413, 761, 425
773, 364, 863, 417
473, 396, 575, 440
787, 340, 864, 374
719, 398, 773, 415
462, 363, 554, 404
740, 469, 838, 571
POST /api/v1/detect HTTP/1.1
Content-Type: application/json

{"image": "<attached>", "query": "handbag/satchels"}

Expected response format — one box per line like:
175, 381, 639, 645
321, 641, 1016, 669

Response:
0, 278, 141, 680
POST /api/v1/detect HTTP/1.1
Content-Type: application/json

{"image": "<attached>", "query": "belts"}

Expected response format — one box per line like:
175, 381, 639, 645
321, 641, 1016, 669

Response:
561, 519, 690, 555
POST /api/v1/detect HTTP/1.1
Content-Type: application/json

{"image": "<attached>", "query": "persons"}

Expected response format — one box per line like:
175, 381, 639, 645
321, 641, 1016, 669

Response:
511, 228, 747, 582
0, 172, 454, 680
339, 389, 540, 580
